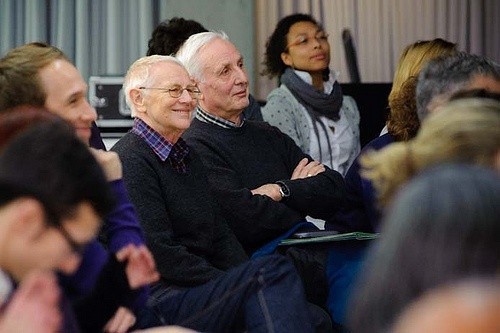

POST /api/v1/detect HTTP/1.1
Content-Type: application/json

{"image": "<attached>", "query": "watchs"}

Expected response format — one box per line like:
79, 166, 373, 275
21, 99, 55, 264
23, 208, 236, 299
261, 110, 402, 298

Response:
274, 180, 291, 200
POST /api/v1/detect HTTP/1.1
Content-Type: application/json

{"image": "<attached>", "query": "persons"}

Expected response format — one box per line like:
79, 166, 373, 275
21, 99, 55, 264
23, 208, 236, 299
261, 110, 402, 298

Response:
0, 17, 500, 333
261, 14, 361, 229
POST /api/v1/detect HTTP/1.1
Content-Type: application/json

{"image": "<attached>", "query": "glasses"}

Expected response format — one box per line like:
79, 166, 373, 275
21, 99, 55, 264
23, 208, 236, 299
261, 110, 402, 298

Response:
290, 32, 331, 51
137, 86, 206, 101
51, 223, 89, 255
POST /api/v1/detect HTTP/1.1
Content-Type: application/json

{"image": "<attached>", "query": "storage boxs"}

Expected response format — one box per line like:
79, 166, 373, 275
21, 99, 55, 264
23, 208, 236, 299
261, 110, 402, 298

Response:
89, 76, 135, 139
